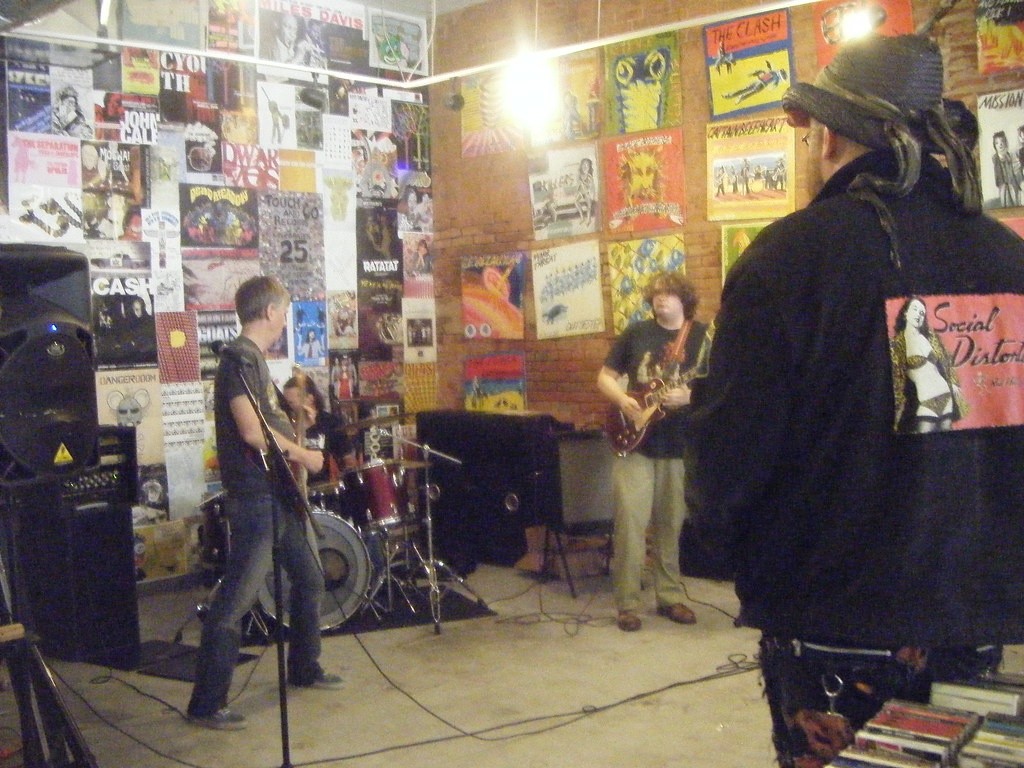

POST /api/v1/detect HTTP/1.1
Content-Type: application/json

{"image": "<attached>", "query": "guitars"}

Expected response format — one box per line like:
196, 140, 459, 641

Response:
280, 362, 310, 523
604, 367, 696, 457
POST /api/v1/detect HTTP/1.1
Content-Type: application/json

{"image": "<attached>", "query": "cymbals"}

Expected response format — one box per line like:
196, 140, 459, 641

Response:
336, 412, 419, 433
383, 459, 434, 470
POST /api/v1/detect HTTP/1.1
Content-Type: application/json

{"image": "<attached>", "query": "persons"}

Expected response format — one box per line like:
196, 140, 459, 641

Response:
686, 35, 1024, 767
126, 296, 146, 322
412, 240, 430, 274
282, 377, 383, 577
81, 144, 105, 188
599, 275, 714, 631
270, 16, 325, 67
185, 275, 345, 731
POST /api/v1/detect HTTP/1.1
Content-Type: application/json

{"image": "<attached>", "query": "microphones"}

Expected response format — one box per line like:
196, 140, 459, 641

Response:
210, 340, 254, 368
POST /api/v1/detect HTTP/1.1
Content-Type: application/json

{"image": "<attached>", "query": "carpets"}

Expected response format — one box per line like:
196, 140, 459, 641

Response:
321, 583, 499, 637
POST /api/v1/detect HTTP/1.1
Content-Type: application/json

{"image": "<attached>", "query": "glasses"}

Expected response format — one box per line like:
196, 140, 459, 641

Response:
802, 124, 820, 146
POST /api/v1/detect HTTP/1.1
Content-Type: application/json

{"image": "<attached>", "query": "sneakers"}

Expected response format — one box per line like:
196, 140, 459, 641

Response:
187, 708, 247, 730
311, 673, 344, 688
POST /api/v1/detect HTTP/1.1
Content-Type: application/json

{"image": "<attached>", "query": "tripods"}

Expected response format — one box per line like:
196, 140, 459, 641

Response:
360, 425, 497, 637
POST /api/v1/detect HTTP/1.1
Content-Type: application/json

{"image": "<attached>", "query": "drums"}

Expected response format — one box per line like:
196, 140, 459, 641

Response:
342, 457, 417, 532
256, 506, 373, 632
307, 479, 347, 515
197, 491, 229, 571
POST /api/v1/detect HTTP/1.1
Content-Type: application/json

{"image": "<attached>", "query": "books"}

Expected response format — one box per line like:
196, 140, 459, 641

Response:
833, 644, 1024, 767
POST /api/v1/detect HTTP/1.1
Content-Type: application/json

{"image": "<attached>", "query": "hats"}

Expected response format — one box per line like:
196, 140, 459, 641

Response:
780, 32, 980, 155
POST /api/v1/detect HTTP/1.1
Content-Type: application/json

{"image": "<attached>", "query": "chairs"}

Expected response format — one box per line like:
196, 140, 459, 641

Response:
541, 427, 615, 599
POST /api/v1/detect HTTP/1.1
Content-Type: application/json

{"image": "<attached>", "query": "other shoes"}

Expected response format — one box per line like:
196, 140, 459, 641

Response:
619, 611, 640, 630
657, 603, 695, 623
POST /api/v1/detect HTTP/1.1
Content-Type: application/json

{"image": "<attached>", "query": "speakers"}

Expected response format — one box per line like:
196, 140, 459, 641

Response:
12, 500, 142, 671
0, 244, 104, 489
535, 432, 618, 539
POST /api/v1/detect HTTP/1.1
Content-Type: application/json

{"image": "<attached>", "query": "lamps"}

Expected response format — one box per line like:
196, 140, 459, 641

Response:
301, 73, 329, 108
447, 78, 465, 109
